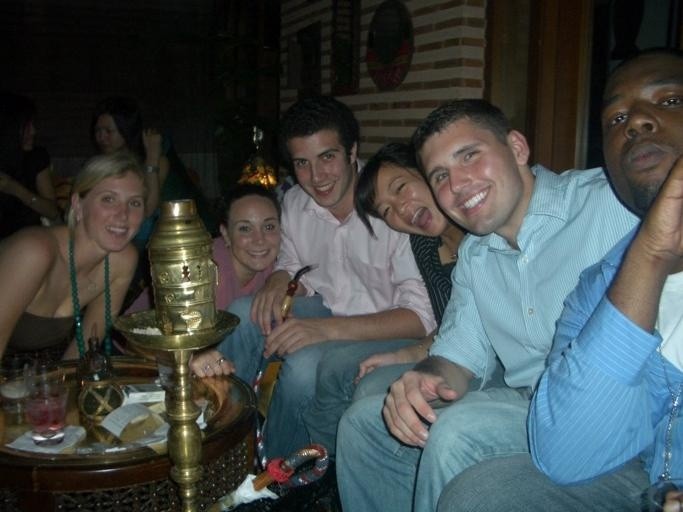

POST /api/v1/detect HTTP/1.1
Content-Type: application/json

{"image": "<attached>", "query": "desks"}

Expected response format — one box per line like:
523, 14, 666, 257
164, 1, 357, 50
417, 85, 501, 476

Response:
0, 353, 260, 510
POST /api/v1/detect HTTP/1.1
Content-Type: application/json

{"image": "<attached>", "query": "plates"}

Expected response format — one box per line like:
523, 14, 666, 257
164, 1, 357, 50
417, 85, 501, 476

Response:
113, 311, 240, 349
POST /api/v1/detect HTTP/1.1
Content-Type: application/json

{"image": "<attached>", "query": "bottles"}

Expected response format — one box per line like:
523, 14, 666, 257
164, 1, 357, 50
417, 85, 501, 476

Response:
81, 336, 109, 381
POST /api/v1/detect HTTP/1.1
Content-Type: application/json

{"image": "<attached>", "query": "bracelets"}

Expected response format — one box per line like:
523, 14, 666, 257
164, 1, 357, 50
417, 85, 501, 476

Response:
30, 194, 40, 206
142, 165, 160, 173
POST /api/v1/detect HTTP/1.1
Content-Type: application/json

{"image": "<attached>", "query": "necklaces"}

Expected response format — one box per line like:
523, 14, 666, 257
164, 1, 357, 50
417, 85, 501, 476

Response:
649, 297, 683, 482
67, 228, 112, 362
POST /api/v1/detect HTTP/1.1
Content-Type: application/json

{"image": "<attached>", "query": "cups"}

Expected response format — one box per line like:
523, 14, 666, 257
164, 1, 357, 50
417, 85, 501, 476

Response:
4, 355, 32, 401
29, 371, 64, 442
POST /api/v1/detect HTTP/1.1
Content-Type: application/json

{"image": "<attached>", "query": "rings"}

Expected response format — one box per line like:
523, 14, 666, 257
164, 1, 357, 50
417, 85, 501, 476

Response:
218, 357, 227, 365
205, 364, 212, 372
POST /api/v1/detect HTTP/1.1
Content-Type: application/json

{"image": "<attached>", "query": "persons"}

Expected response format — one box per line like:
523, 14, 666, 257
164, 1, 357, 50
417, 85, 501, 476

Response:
329, 98, 645, 512
430, 39, 683, 512
0, 95, 59, 239
120, 181, 282, 323
0, 150, 151, 364
340, 139, 477, 407
83, 97, 189, 244
214, 95, 435, 463
